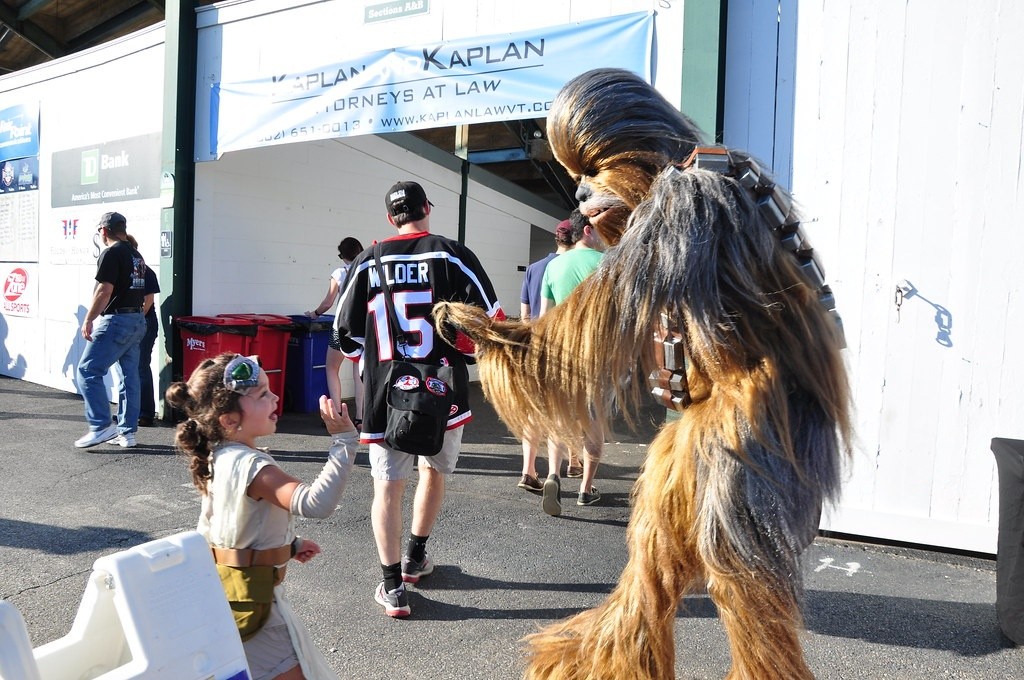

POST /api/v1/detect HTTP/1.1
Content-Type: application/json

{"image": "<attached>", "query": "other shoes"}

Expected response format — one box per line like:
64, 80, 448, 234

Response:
113, 414, 153, 427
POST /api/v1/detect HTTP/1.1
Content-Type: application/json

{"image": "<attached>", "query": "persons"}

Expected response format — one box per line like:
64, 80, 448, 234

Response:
334, 179, 507, 618
112, 233, 160, 428
309, 236, 365, 432
73, 212, 145, 448
538, 207, 602, 515
519, 219, 584, 492
165, 351, 360, 680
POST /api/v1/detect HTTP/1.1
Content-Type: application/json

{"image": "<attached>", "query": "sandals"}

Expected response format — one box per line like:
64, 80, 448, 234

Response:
352, 417, 362, 431
321, 412, 342, 427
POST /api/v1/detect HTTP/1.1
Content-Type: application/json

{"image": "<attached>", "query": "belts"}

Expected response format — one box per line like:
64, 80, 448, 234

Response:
210, 545, 291, 568
104, 307, 143, 313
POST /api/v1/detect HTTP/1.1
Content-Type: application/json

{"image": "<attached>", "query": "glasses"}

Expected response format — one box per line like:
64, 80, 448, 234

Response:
338, 253, 344, 259
224, 353, 263, 396
98, 227, 102, 233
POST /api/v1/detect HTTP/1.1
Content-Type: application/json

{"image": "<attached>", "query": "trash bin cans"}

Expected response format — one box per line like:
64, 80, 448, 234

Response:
175, 316, 258, 384
217, 313, 294, 417
285, 314, 336, 414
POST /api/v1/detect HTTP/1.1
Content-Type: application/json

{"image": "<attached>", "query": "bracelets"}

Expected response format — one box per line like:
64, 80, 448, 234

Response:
315, 309, 320, 317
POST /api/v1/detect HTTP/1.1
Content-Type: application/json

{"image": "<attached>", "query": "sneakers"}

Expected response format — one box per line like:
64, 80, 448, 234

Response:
106, 431, 137, 447
73, 420, 118, 448
566, 459, 584, 477
517, 473, 544, 491
542, 473, 561, 516
401, 551, 433, 583
576, 483, 601, 506
375, 579, 411, 617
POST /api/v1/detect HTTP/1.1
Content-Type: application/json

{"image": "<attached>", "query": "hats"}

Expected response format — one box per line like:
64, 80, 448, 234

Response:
99, 212, 126, 231
556, 220, 574, 240
385, 181, 434, 216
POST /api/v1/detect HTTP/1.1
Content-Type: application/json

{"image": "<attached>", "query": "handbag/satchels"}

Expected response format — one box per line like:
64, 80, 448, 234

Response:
383, 359, 454, 456
